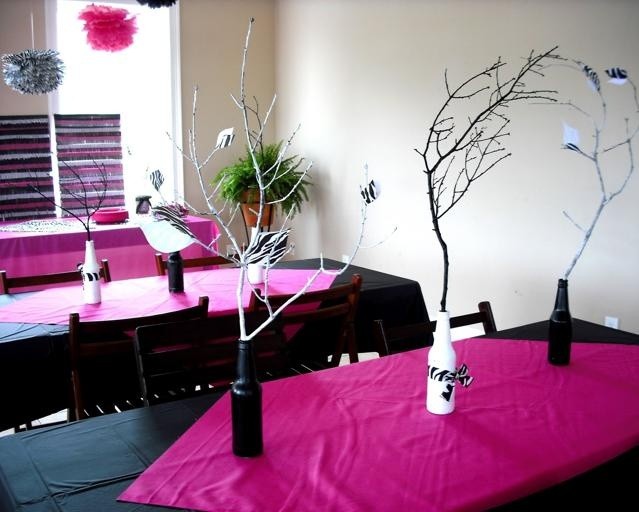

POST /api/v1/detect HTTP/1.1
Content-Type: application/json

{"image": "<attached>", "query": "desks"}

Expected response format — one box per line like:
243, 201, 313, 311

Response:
0, 317, 639, 512
0, 215, 215, 294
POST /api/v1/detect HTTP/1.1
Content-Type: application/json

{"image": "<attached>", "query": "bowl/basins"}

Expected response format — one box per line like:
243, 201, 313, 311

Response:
91, 208, 129, 224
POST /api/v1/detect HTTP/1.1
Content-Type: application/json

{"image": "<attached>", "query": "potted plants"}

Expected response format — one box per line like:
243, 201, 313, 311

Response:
207, 139, 316, 228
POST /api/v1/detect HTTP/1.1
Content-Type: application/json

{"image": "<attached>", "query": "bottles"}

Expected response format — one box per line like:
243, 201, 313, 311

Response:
426, 311, 455, 415
83, 240, 101, 304
168, 250, 184, 292
547, 278, 573, 366
230, 338, 263, 457
248, 225, 263, 284
